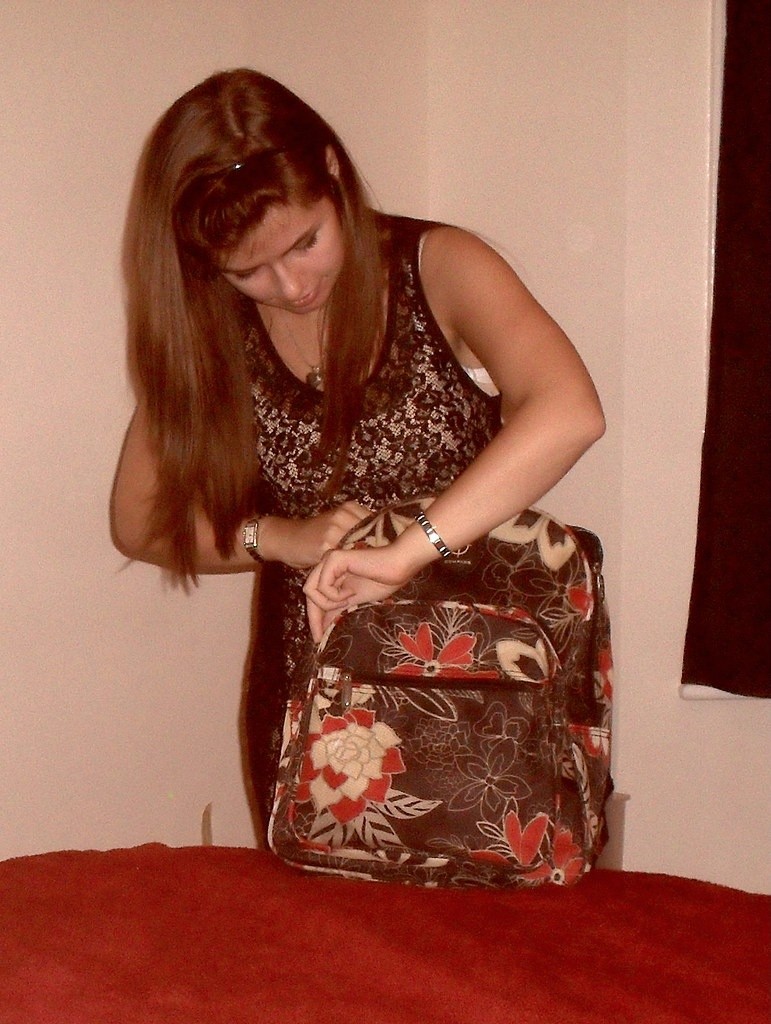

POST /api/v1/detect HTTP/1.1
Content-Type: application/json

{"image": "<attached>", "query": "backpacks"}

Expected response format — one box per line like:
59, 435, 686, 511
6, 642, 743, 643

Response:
269, 498, 612, 892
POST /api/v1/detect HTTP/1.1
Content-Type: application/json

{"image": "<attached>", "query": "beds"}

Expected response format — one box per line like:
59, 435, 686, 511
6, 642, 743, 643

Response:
0, 842, 771, 1024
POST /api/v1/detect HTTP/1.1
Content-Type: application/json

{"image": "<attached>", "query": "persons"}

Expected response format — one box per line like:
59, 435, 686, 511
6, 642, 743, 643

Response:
107, 68, 609, 852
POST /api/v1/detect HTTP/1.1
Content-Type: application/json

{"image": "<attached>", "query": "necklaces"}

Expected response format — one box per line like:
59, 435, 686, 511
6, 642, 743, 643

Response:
276, 306, 326, 390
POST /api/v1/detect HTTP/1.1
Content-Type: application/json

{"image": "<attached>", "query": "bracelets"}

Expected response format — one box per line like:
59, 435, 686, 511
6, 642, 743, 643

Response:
414, 510, 455, 562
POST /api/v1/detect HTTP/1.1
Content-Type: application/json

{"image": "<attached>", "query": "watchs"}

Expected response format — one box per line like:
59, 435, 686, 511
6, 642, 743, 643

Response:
241, 513, 271, 564
